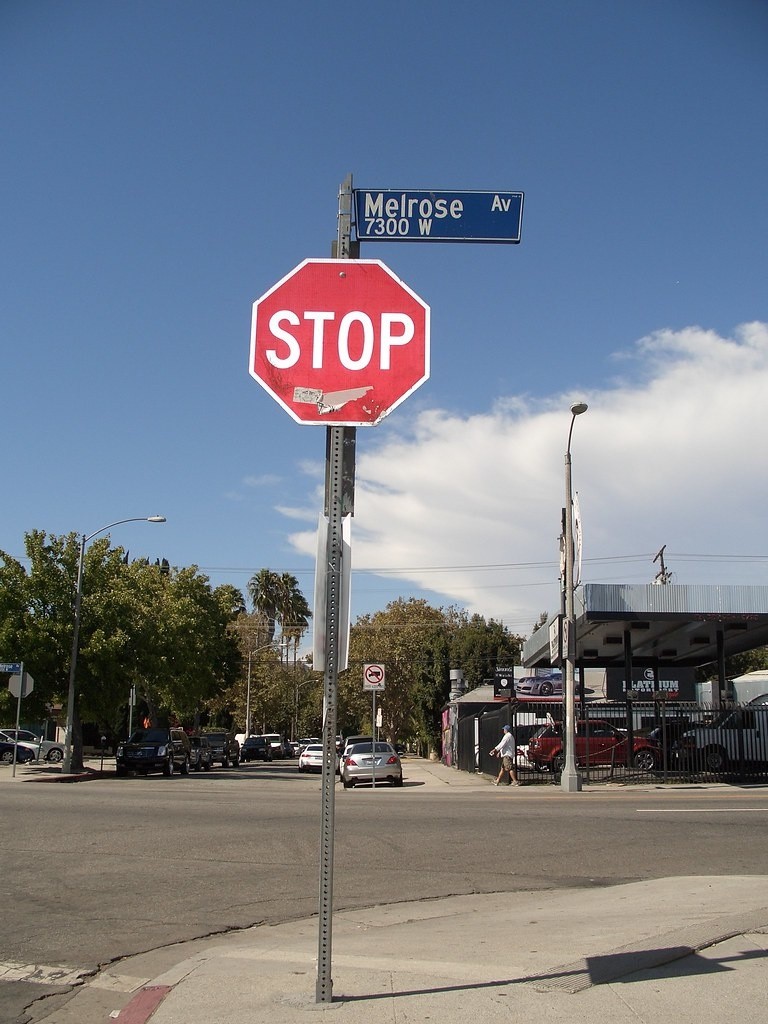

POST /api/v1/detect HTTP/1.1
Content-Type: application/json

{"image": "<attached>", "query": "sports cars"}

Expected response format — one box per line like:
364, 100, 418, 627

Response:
517, 674, 580, 697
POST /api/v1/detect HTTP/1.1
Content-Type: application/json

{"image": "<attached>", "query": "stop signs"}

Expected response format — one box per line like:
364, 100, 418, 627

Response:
244, 259, 434, 433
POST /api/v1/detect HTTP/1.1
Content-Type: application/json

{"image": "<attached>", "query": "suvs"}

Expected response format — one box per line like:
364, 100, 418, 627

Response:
203, 731, 240, 768
527, 720, 662, 772
116, 725, 191, 776
683, 708, 768, 772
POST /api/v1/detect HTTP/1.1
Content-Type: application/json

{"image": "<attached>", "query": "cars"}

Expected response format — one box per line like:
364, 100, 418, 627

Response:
514, 745, 549, 773
0, 728, 65, 763
284, 726, 404, 788
649, 722, 706, 770
188, 736, 212, 772
240, 737, 272, 764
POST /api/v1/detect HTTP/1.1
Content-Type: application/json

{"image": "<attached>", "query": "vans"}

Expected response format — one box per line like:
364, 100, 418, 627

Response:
261, 733, 284, 760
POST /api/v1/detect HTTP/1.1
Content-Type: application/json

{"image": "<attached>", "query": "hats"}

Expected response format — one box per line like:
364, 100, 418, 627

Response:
502, 725, 511, 730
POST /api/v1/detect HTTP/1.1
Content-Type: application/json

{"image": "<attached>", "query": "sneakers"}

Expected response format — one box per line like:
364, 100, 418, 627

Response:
509, 781, 519, 787
491, 780, 499, 786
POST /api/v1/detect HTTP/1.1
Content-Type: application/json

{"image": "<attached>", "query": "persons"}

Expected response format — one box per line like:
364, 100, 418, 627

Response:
490, 725, 520, 787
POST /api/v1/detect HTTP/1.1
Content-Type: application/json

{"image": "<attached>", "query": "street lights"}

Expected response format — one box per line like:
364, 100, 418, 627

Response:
245, 642, 287, 742
560, 400, 592, 797
62, 513, 167, 775
